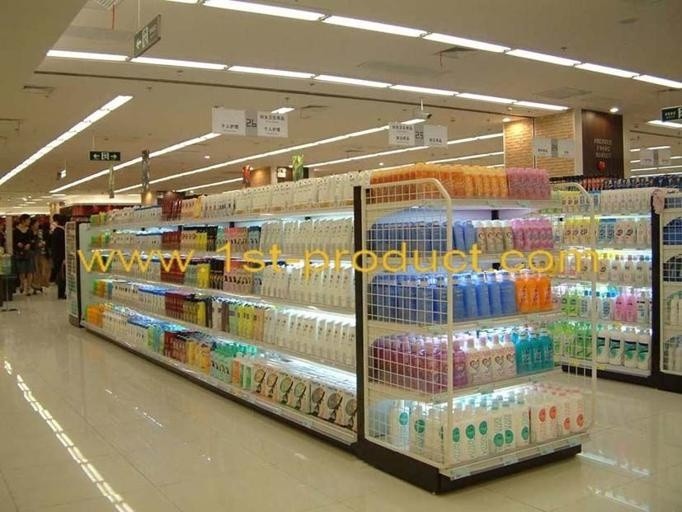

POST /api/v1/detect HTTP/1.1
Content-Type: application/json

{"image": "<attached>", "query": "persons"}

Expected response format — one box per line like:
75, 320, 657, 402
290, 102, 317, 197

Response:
13, 212, 66, 300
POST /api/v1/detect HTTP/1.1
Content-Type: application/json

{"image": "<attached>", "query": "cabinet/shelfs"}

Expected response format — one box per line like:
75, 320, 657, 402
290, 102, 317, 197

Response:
65, 177, 682, 496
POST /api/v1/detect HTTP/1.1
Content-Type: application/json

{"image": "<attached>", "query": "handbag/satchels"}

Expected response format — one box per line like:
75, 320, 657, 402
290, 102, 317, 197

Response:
16, 251, 30, 260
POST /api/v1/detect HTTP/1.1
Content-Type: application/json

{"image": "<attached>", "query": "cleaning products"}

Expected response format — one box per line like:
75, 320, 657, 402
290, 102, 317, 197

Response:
81, 164, 682, 464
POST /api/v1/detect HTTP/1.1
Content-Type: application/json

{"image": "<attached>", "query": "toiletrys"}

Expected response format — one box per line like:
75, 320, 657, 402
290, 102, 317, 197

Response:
81, 164, 682, 464
